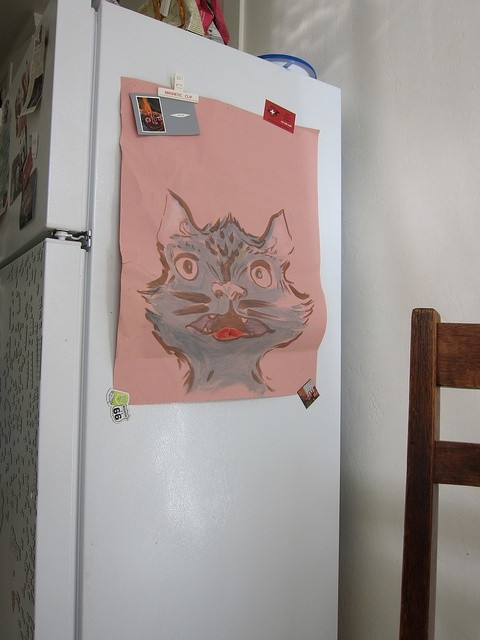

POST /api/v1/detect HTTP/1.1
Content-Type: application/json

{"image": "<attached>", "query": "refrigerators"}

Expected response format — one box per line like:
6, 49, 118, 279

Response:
0, 1, 341, 640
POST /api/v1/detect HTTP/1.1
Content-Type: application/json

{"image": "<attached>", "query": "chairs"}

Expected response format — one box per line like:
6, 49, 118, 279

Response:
397, 306, 479, 639
